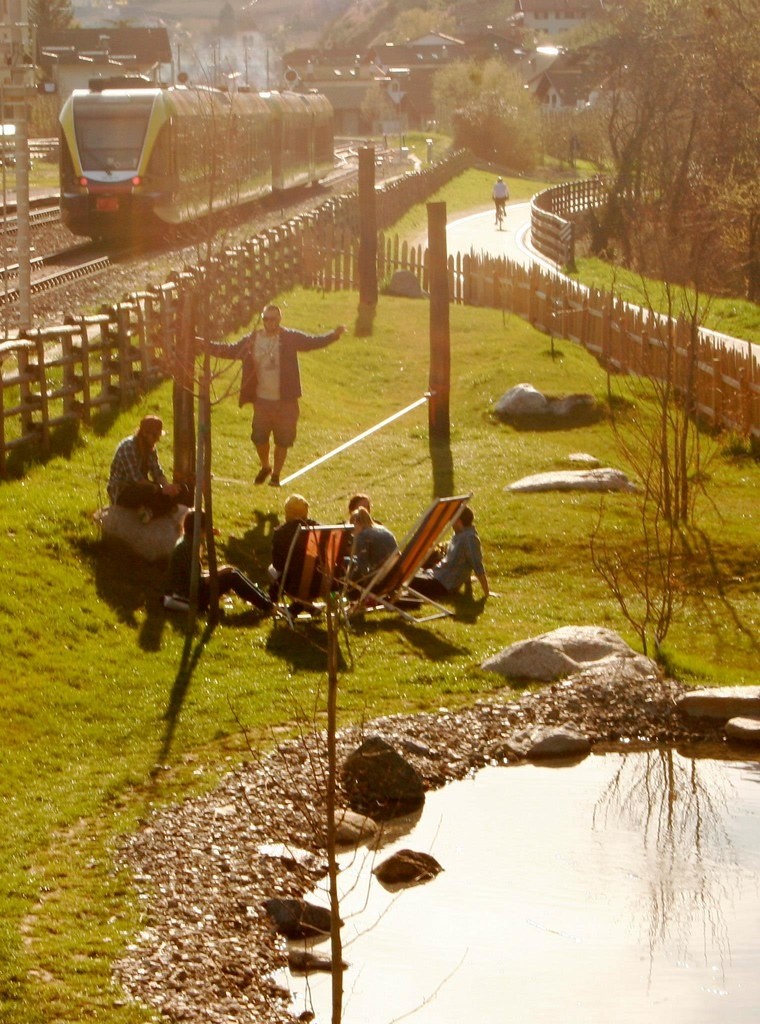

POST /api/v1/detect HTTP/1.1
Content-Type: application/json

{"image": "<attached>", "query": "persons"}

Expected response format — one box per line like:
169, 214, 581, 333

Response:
267, 492, 501, 605
193, 305, 348, 488
107, 415, 194, 526
492, 175, 510, 225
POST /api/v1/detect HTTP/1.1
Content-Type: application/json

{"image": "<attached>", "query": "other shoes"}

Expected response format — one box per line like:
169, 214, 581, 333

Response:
269, 473, 281, 487
256, 466, 271, 483
139, 507, 153, 523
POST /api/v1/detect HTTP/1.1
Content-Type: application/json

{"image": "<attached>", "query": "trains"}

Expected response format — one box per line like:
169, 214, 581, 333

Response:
58, 75, 335, 246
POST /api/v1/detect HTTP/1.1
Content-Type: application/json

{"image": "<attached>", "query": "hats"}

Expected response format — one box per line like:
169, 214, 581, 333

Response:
284, 494, 309, 520
139, 417, 163, 434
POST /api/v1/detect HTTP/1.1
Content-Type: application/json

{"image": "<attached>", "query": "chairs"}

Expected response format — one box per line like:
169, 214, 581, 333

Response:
271, 522, 356, 637
349, 491, 474, 622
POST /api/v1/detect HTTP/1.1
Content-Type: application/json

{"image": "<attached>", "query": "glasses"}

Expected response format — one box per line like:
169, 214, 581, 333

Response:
262, 316, 277, 322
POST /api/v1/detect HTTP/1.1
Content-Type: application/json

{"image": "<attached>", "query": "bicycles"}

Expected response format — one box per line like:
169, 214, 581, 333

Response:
495, 202, 506, 230
